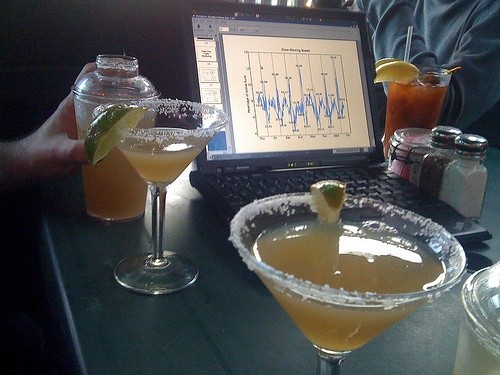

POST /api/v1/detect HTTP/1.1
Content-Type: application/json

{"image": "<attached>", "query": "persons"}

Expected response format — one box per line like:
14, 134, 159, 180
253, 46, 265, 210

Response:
0, 62, 105, 189
306, 0, 500, 149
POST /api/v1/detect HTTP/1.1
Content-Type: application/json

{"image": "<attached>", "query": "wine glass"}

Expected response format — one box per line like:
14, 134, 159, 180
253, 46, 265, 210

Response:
92, 98, 230, 295
230, 191, 468, 375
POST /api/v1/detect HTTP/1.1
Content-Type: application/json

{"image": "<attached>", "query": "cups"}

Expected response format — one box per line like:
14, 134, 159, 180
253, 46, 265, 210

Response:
382, 65, 452, 166
453, 261, 500, 375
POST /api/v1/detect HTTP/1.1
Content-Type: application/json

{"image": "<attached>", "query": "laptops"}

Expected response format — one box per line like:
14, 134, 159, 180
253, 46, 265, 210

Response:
175, 0, 492, 244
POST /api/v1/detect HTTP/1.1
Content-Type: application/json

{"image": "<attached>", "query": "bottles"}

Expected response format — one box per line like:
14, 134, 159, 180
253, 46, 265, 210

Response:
437, 133, 488, 221
387, 128, 432, 187
418, 125, 463, 198
71, 55, 161, 223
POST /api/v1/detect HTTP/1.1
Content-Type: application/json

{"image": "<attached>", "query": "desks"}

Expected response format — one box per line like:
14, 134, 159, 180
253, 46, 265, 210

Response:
39, 144, 500, 375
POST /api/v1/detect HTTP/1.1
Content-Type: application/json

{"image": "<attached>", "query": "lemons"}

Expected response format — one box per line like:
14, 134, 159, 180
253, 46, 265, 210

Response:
311, 178, 345, 224
373, 58, 419, 84
84, 103, 143, 167
